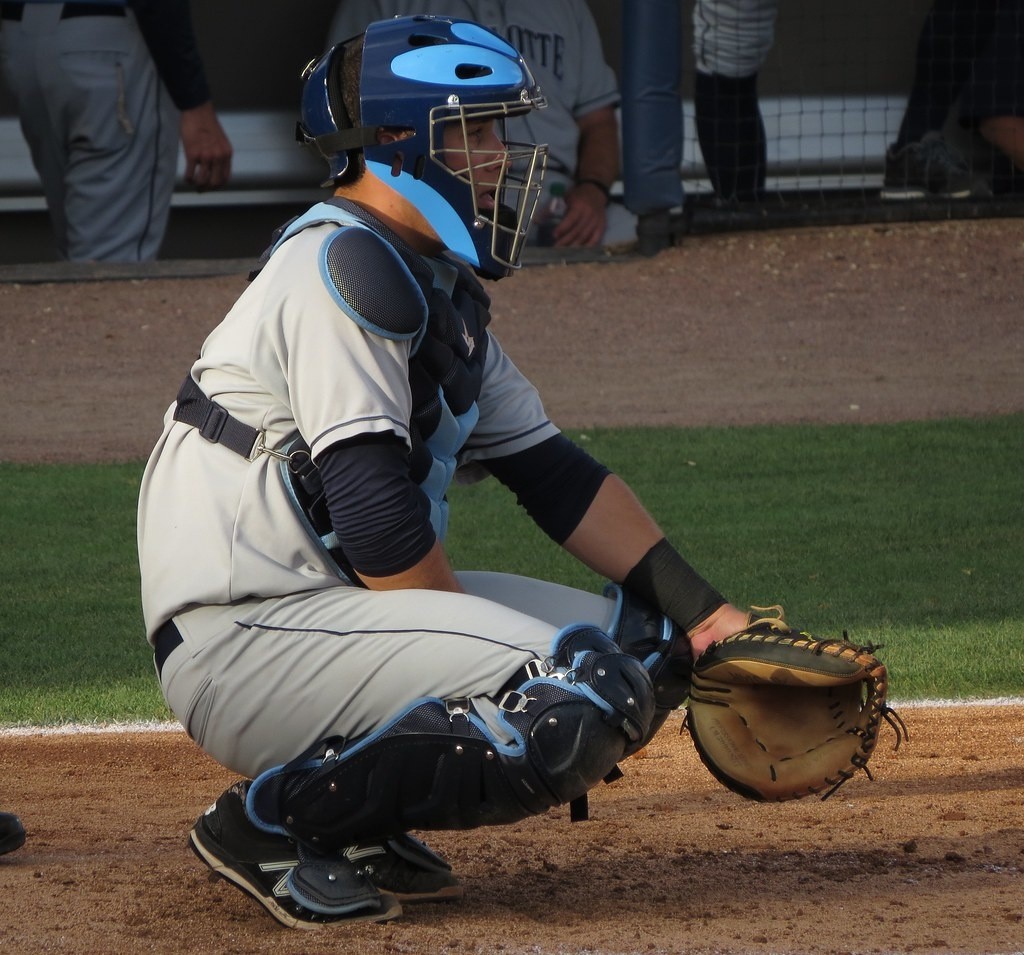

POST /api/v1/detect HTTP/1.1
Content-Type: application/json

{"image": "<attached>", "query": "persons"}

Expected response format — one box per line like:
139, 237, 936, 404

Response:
2, 1, 235, 272
125, 6, 747, 936
318, 1, 1023, 265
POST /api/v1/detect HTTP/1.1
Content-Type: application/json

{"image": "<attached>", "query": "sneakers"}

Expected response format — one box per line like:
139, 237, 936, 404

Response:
183, 779, 401, 932
328, 830, 465, 908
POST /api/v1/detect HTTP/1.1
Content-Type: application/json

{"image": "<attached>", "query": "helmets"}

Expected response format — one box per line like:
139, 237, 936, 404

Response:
290, 10, 551, 283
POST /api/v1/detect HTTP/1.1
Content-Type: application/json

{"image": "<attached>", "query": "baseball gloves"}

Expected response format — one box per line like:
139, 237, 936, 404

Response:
687, 604, 909, 801
